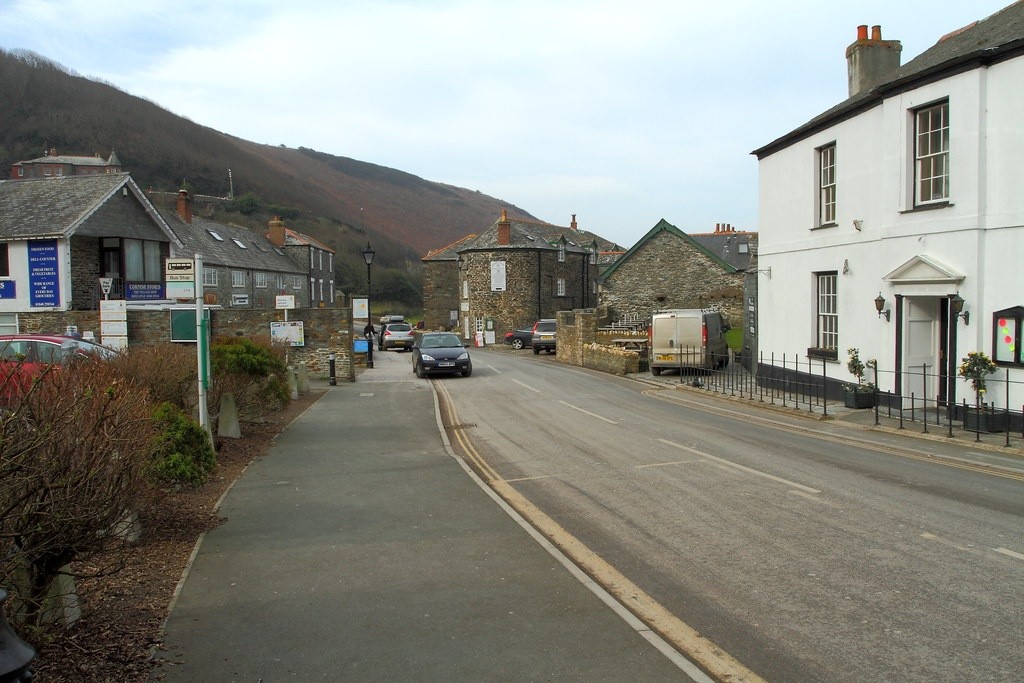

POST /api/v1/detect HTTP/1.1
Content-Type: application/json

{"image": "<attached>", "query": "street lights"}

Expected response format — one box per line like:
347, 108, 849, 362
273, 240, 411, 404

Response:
362, 239, 376, 369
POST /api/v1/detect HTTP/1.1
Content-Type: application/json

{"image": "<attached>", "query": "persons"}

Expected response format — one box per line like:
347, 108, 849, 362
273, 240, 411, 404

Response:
364, 321, 379, 351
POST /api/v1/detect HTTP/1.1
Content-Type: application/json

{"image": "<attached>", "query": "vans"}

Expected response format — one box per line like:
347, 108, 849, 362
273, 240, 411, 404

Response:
648, 306, 732, 375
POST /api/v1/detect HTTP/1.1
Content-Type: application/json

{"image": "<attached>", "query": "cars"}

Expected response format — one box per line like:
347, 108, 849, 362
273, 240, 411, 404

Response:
0, 333, 127, 403
503, 325, 535, 349
410, 331, 473, 378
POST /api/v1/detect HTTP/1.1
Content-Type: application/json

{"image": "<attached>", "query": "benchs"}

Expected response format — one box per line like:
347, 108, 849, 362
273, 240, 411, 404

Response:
625, 350, 643, 352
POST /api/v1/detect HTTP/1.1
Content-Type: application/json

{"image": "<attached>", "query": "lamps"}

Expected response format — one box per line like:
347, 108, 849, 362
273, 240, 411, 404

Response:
874, 292, 890, 322
950, 291, 969, 325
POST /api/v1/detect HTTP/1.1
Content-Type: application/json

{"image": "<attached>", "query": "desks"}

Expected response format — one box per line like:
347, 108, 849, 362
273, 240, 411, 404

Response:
612, 338, 648, 359
598, 320, 646, 332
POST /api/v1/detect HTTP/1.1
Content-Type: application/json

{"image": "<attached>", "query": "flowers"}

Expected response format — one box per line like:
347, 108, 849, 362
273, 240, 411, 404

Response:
957, 352, 998, 409
842, 348, 874, 392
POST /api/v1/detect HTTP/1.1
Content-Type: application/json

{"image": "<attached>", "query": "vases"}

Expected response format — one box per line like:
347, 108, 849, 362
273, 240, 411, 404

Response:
844, 389, 874, 409
965, 404, 1006, 434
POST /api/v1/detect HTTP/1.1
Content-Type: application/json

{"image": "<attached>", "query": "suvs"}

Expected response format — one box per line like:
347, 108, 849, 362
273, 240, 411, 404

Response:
530, 319, 558, 354
374, 315, 415, 351
